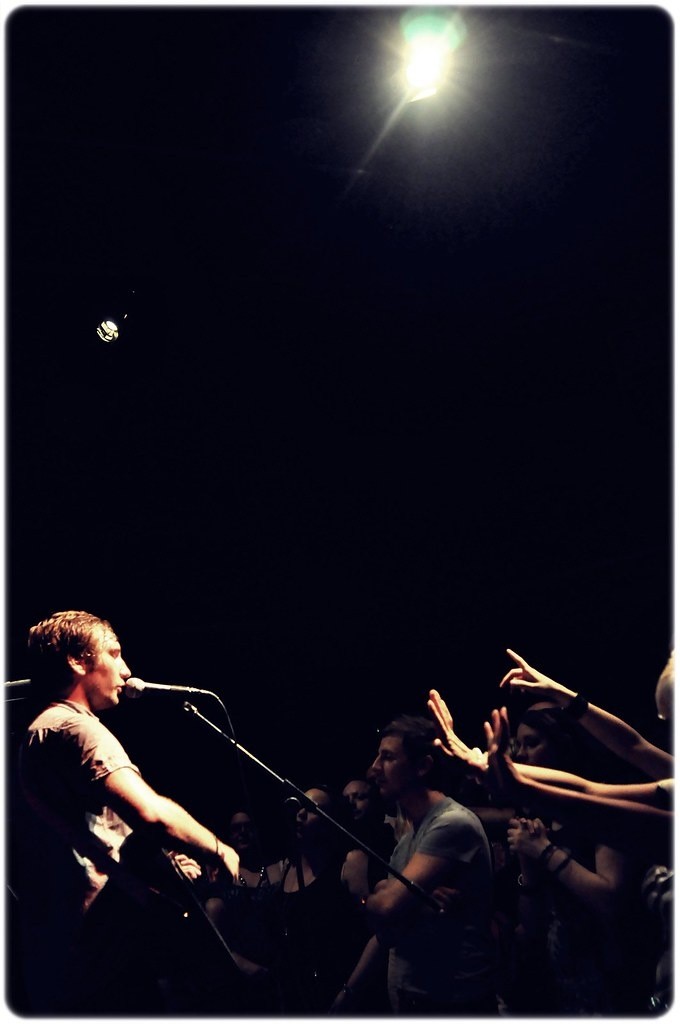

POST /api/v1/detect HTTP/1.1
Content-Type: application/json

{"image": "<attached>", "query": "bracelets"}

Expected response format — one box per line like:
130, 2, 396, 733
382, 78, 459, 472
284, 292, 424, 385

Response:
551, 856, 573, 882
538, 844, 559, 869
359, 895, 369, 912
562, 694, 589, 719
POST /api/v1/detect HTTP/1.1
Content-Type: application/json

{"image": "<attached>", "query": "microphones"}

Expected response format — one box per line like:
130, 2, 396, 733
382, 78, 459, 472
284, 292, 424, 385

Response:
125, 678, 211, 699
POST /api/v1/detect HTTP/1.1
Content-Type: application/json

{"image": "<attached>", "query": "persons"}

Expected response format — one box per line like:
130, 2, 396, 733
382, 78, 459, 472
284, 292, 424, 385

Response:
19, 611, 672, 1016
